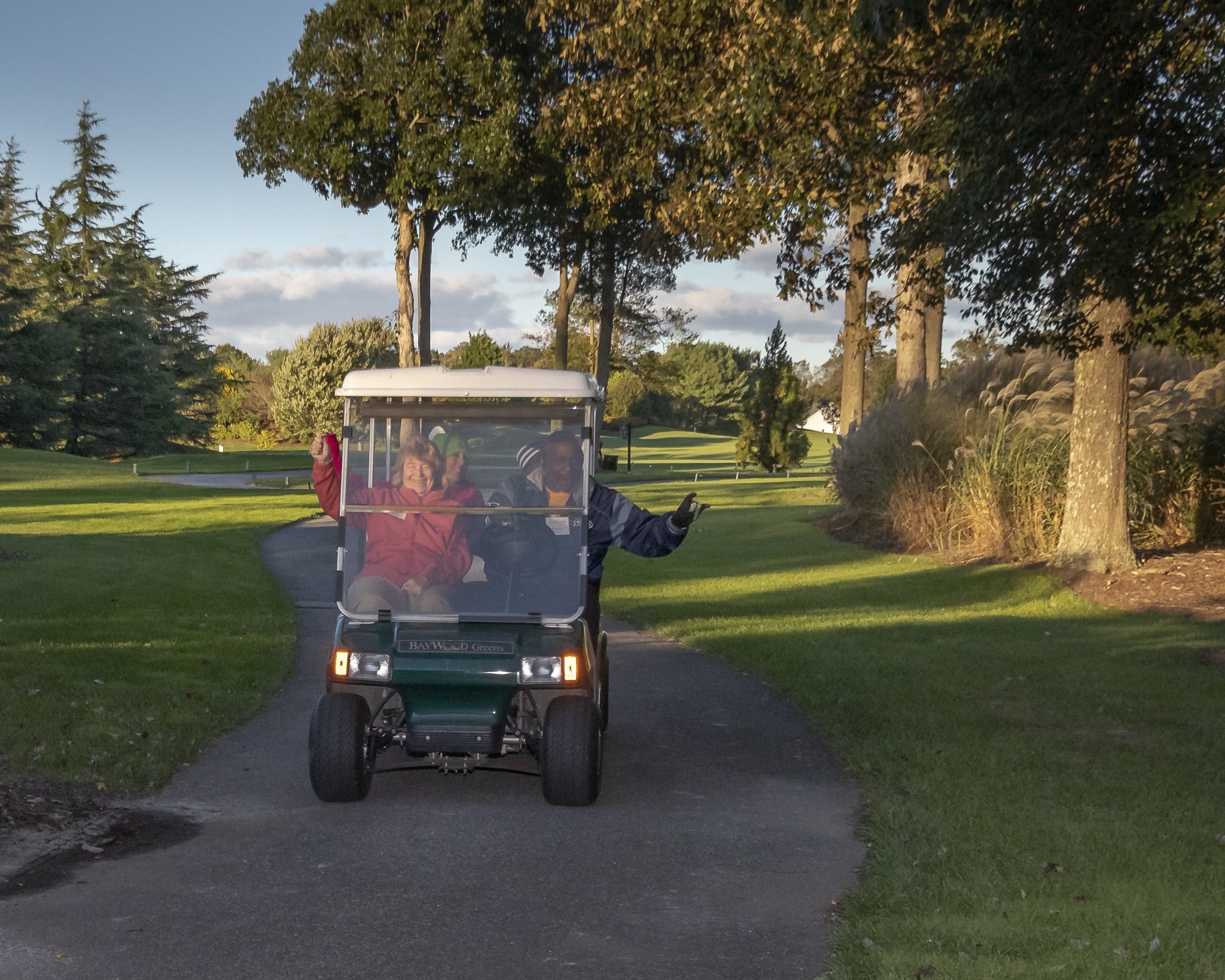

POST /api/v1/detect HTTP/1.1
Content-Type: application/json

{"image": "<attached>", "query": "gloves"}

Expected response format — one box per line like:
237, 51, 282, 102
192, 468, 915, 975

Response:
665, 492, 711, 535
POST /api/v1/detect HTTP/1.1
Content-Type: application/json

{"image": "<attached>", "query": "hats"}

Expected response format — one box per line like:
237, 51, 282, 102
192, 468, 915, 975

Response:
433, 433, 471, 462
515, 440, 545, 470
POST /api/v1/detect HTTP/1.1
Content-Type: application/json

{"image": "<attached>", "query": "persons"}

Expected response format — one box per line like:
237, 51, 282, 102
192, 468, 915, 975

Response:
317, 432, 486, 530
308, 430, 475, 618
487, 441, 549, 524
465, 431, 710, 658
